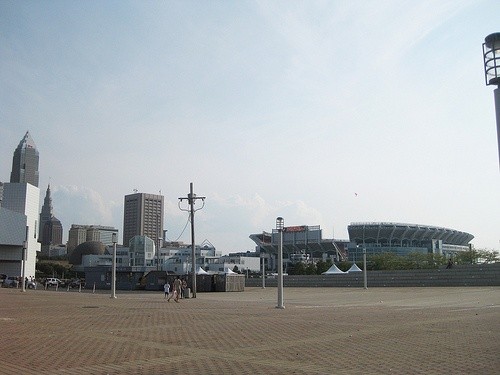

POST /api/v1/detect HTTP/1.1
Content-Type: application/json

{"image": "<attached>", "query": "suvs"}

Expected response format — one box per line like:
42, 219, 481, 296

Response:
41, 278, 86, 289
5, 276, 36, 289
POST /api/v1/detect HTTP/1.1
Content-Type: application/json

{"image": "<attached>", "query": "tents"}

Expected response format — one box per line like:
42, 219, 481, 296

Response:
346, 263, 362, 271
220, 268, 237, 275
196, 267, 209, 275
320, 264, 347, 274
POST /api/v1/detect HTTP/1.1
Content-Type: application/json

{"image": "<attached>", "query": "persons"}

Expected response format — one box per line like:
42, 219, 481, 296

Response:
164, 282, 170, 298
173, 276, 182, 302
30, 275, 33, 282
181, 278, 184, 299
25, 277, 28, 287
33, 276, 36, 283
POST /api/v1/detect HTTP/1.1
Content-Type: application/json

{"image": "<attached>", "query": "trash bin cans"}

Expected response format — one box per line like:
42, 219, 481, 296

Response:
184, 287, 190, 298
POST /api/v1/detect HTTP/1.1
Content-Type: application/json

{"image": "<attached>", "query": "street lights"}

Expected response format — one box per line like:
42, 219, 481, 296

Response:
275, 216, 285, 309
356, 242, 360, 265
158, 237, 164, 271
21, 240, 28, 292
482, 31, 500, 172
111, 232, 119, 299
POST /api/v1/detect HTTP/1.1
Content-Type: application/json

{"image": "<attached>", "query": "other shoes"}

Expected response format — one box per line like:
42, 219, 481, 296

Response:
174, 299, 180, 303
